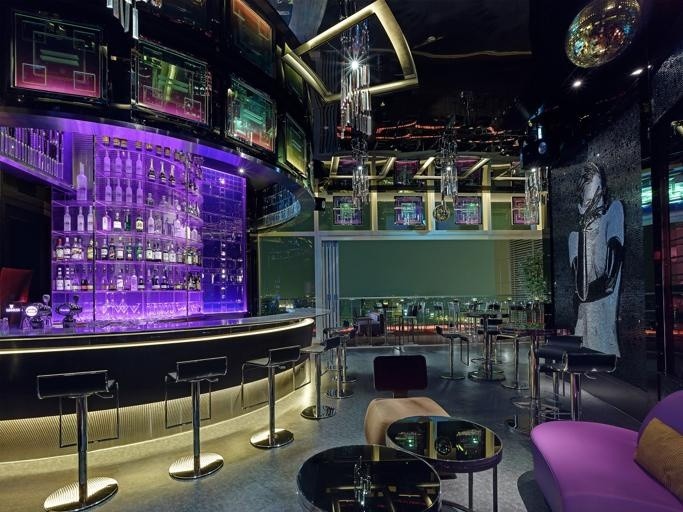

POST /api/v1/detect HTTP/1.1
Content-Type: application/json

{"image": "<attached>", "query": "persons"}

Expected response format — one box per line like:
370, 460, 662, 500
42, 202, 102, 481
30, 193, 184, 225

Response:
568, 161, 625, 380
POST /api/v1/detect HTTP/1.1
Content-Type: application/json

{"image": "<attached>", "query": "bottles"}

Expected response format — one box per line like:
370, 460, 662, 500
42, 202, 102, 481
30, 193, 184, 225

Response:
75, 162, 87, 200
102, 137, 201, 205
52, 206, 202, 292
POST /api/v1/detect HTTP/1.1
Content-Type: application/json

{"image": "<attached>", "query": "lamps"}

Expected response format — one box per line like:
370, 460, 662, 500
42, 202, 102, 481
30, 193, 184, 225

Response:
335, 0, 646, 232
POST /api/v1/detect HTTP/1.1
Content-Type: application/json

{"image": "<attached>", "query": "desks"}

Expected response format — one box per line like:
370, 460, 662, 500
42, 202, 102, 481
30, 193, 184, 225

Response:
382, 415, 503, 512
291, 443, 444, 511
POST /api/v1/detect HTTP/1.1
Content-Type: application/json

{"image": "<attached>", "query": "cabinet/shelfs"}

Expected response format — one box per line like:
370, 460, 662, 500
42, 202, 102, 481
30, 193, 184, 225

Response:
49, 144, 204, 323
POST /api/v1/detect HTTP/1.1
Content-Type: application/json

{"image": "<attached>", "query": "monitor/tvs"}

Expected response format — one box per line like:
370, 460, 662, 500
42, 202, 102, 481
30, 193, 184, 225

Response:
9, 9, 109, 107
221, 67, 279, 162
285, 112, 317, 194
131, 35, 213, 129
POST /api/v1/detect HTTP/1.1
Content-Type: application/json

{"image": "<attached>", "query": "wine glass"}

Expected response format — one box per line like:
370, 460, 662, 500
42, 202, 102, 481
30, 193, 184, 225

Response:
0, 293, 201, 336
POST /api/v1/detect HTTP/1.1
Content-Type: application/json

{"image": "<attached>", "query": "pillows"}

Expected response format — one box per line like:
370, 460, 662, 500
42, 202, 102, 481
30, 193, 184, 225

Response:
632, 416, 683, 505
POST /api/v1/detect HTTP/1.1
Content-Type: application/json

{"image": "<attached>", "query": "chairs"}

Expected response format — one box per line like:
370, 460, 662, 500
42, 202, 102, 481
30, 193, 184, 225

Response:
31, 366, 124, 511
362, 354, 473, 511
296, 336, 340, 420
159, 356, 229, 480
240, 343, 302, 449
434, 309, 618, 437
265, 293, 545, 349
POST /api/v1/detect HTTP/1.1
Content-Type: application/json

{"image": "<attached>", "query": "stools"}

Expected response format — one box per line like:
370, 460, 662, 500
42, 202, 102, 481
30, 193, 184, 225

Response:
322, 320, 358, 398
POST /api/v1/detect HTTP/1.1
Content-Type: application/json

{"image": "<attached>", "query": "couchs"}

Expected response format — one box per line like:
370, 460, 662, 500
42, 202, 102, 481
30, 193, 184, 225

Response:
528, 389, 682, 512
